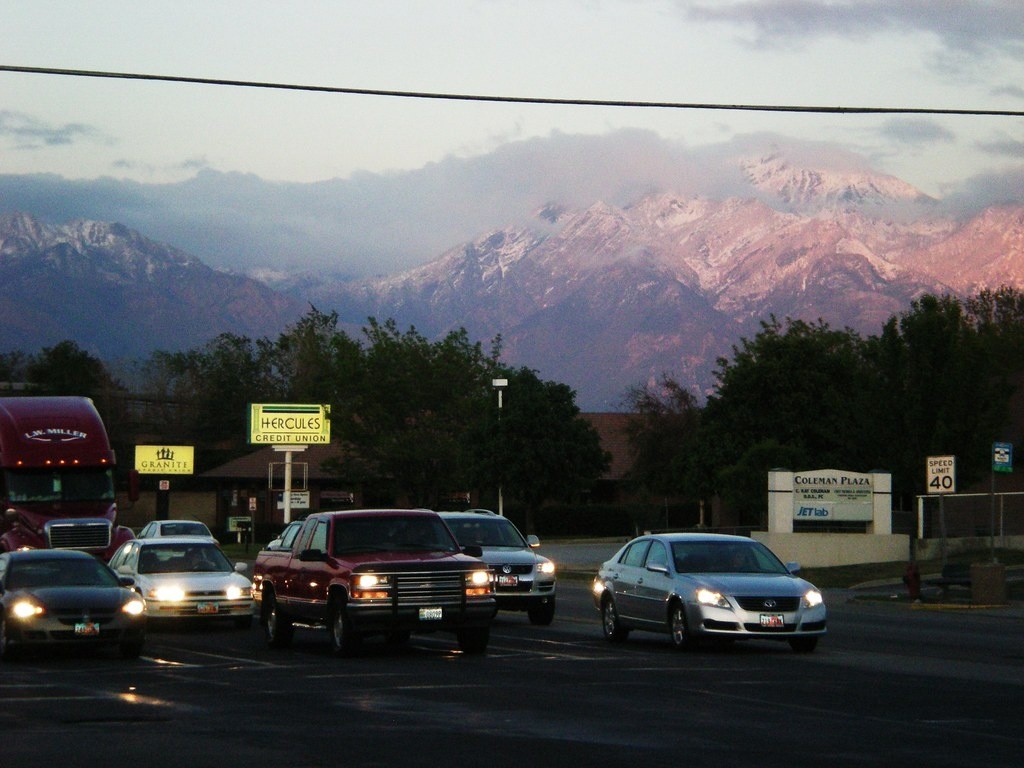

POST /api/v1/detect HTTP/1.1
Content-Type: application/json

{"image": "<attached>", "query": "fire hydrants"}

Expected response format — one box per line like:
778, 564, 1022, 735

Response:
902, 564, 923, 602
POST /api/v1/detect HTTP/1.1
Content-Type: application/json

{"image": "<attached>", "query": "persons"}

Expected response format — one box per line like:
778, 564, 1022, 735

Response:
403, 522, 432, 547
189, 552, 216, 571
728, 551, 756, 571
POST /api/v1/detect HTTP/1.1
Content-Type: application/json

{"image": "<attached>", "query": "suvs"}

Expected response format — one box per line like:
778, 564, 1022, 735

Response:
434, 508, 558, 627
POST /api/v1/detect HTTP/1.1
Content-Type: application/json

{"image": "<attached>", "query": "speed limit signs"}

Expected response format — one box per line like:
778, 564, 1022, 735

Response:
926, 456, 956, 494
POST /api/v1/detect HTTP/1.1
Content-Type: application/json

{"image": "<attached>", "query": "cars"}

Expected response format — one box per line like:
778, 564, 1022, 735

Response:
592, 532, 828, 654
1, 549, 146, 664
108, 536, 257, 633
139, 520, 220, 562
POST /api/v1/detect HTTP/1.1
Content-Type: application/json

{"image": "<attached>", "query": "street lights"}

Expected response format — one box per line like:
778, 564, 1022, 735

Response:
492, 379, 509, 518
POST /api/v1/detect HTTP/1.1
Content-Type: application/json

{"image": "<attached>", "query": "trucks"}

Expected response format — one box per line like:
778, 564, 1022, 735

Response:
1, 395, 138, 563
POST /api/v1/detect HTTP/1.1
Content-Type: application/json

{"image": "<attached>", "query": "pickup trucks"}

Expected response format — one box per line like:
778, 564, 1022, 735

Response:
250, 508, 500, 657
